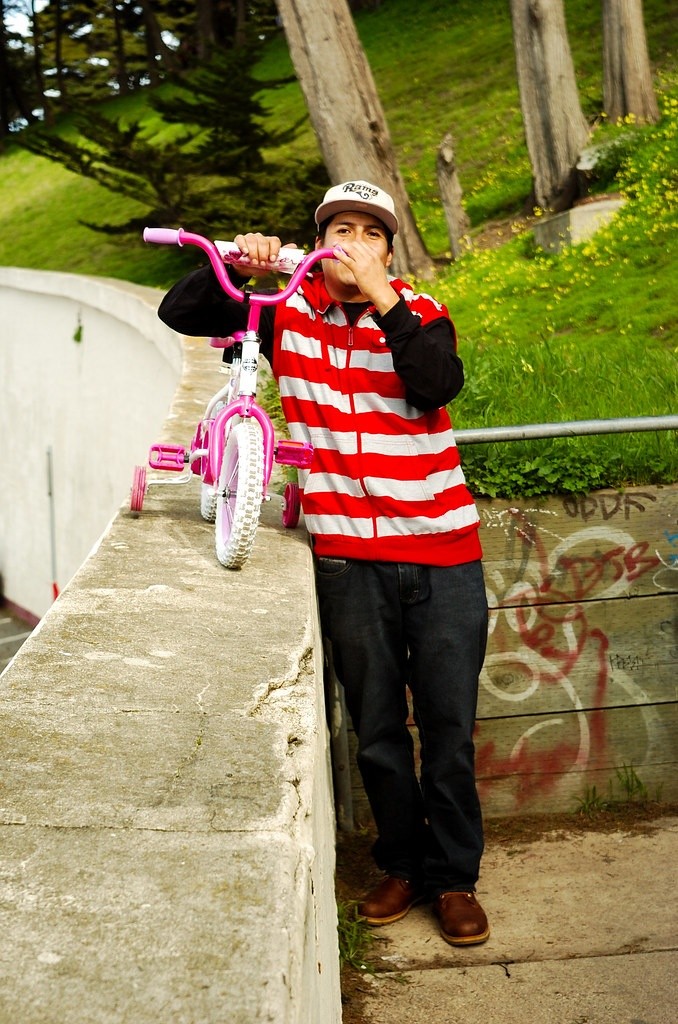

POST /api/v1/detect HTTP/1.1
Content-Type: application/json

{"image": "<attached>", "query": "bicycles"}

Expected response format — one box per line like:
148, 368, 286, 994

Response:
128, 224, 363, 570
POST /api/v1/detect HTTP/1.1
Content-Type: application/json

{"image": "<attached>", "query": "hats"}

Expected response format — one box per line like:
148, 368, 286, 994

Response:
314, 180, 399, 234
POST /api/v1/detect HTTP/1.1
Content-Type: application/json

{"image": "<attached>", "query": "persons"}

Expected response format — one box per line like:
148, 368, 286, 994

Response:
155, 178, 496, 950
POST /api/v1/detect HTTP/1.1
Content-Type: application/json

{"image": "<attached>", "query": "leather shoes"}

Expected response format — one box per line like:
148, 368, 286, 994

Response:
356, 876, 425, 923
436, 891, 490, 947
